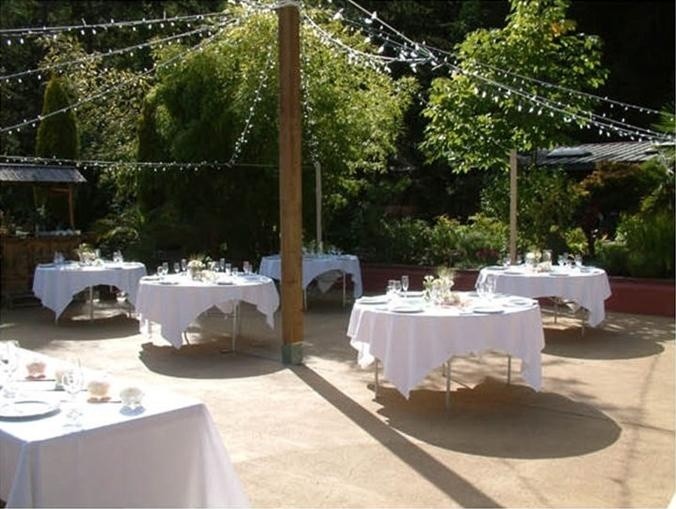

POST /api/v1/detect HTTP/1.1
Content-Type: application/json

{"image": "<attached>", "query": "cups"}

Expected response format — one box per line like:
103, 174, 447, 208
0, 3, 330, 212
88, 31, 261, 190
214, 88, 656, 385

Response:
52, 250, 123, 267
0, 340, 143, 410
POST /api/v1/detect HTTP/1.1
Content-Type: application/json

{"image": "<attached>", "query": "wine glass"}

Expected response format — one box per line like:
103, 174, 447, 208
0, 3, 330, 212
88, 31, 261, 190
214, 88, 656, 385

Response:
502, 250, 586, 277
387, 273, 495, 304
156, 259, 253, 284
62, 358, 85, 427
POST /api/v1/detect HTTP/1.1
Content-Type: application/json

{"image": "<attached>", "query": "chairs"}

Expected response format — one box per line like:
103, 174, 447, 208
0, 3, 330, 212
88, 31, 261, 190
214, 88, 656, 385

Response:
0, 233, 82, 310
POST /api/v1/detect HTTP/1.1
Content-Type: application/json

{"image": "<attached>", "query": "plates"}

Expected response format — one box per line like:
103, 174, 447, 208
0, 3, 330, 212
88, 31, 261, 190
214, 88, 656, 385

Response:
0, 394, 59, 419
473, 307, 503, 314
504, 298, 540, 304
358, 297, 390, 304
391, 306, 422, 313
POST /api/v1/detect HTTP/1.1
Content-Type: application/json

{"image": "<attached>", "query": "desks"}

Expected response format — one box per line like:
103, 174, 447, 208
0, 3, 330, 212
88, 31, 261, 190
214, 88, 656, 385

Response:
3, 340, 253, 506
30, 260, 148, 327
346, 289, 551, 408
133, 270, 281, 351
258, 252, 365, 312
475, 261, 613, 341
